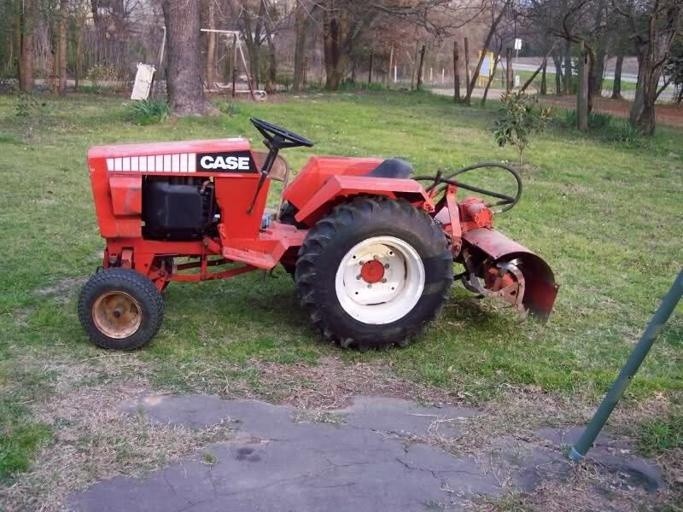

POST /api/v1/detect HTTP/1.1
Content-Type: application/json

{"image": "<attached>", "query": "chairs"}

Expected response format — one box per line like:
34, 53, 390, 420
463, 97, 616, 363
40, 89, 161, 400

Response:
351, 160, 413, 179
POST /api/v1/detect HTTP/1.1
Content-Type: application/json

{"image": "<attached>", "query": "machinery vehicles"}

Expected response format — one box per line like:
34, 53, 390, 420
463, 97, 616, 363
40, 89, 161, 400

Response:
73, 116, 562, 353
